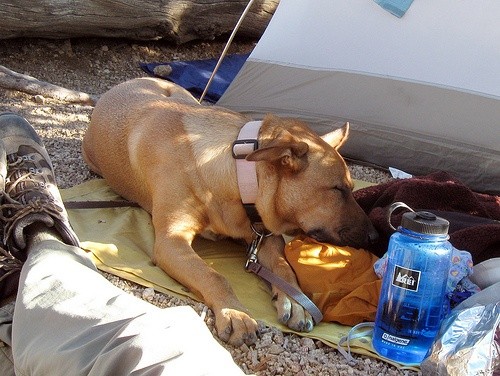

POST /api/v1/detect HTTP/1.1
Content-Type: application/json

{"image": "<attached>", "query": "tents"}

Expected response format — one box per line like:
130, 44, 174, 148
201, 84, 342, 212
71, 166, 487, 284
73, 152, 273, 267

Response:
197, 0, 500, 198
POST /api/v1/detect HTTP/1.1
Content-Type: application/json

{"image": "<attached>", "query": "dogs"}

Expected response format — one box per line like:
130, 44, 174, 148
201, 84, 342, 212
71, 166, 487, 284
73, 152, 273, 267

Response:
80, 75, 379, 349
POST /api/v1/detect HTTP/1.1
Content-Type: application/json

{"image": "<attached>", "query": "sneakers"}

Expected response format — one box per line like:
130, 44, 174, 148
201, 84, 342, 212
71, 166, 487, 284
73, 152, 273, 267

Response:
0, 247, 24, 300
0, 114, 80, 256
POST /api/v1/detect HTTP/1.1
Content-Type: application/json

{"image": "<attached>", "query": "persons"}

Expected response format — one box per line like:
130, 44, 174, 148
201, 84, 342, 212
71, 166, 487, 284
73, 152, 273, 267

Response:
0, 111, 245, 376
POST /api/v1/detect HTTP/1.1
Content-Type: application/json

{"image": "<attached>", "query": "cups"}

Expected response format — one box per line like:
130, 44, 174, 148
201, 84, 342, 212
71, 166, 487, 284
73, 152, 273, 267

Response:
372, 202, 453, 364
